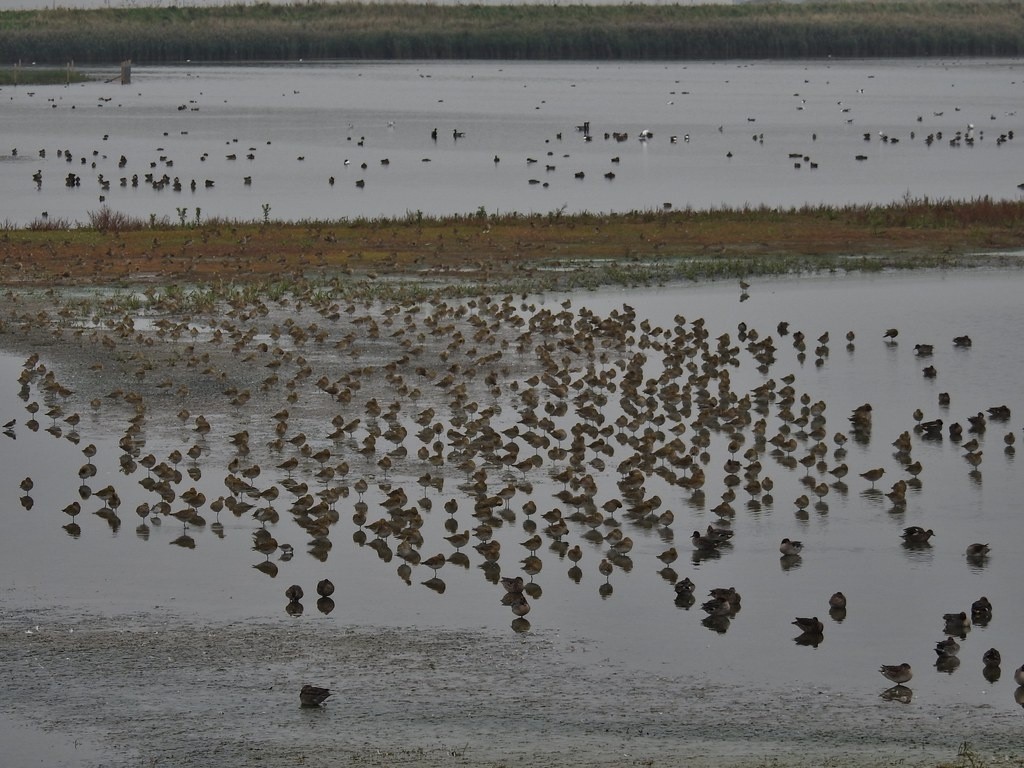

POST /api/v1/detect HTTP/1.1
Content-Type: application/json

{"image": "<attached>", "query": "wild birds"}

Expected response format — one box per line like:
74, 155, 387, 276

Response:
0, 70, 1024, 712
